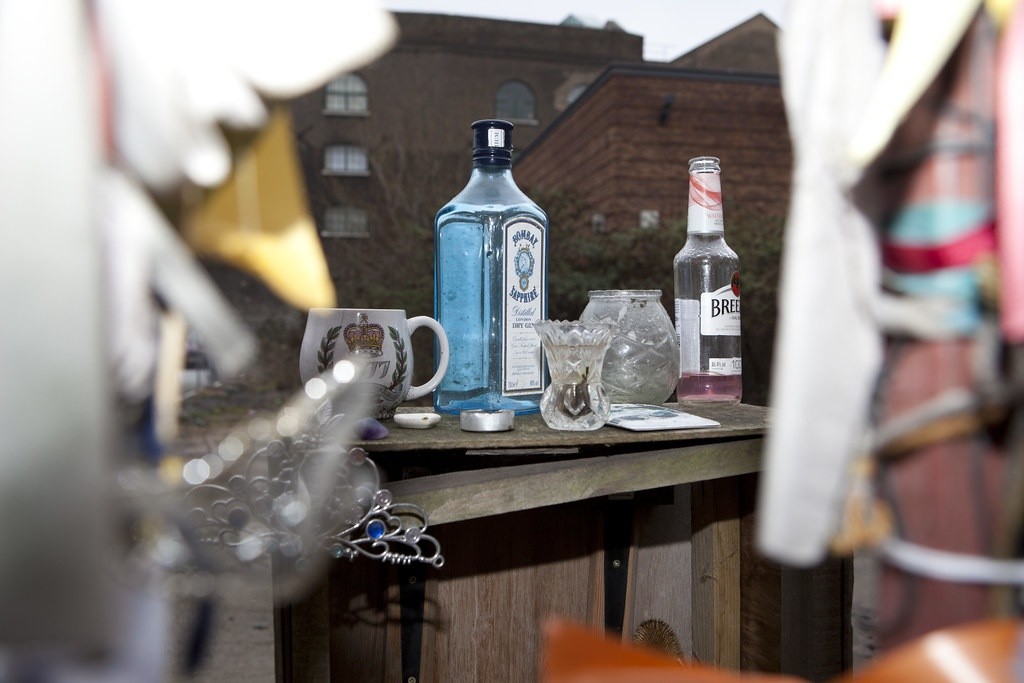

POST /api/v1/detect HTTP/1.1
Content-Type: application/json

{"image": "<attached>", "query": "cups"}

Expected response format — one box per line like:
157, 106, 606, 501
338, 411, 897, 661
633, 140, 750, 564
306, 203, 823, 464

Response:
299, 308, 449, 418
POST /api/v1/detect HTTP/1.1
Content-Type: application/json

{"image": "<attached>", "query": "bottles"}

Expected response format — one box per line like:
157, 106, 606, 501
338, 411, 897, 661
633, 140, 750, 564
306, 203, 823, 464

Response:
531, 319, 618, 430
579, 289, 681, 406
433, 119, 550, 415
674, 155, 742, 407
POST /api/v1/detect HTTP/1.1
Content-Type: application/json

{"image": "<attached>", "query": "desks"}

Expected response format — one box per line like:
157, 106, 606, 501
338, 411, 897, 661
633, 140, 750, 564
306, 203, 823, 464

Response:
268, 402, 857, 683
